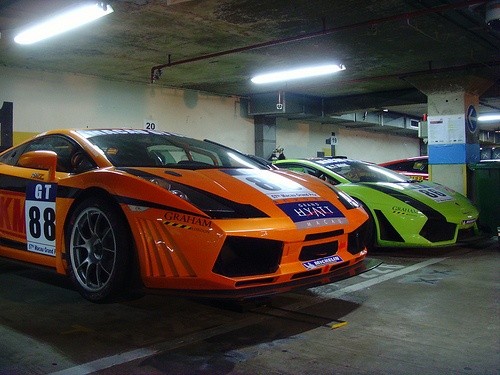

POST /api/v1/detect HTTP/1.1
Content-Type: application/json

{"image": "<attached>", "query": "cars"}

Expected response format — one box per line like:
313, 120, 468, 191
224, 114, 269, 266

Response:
377, 155, 428, 181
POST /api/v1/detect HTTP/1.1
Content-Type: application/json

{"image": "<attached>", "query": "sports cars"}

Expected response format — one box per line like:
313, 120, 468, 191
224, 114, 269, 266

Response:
0, 128, 370, 302
271, 158, 480, 251
